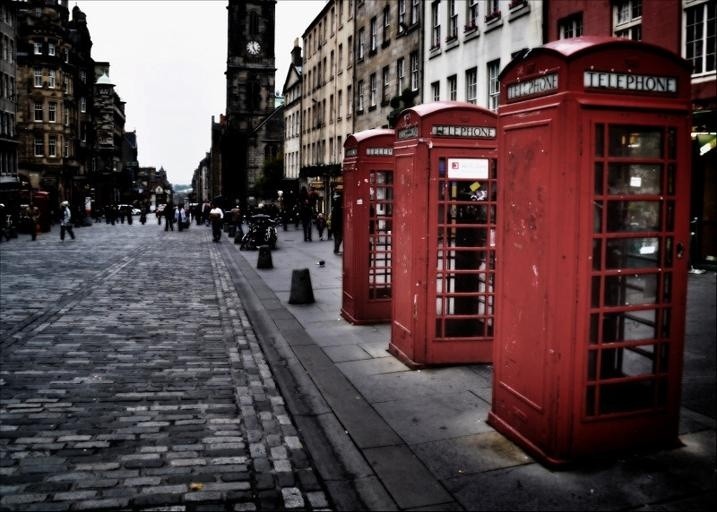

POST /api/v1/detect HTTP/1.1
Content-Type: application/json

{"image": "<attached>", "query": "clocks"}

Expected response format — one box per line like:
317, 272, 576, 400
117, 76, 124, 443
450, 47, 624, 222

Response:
245, 39, 263, 56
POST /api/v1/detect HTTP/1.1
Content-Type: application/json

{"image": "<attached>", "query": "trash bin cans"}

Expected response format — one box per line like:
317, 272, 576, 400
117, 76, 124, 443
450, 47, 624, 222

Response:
223, 223, 229, 232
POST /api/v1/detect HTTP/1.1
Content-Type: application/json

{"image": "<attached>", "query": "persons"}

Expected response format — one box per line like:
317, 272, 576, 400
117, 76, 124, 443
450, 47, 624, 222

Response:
278, 193, 345, 255
25, 201, 42, 243
92, 194, 245, 245
0, 203, 11, 242
59, 200, 76, 243
